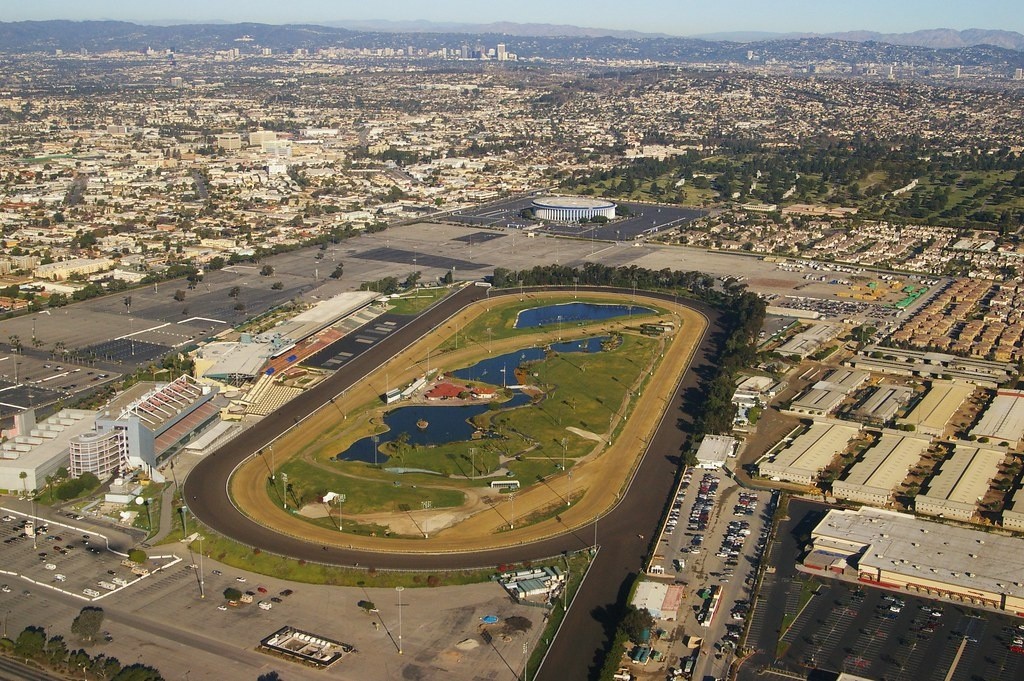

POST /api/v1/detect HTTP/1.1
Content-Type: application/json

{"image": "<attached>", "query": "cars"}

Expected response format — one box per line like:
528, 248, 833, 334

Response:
0, 513, 149, 595
787, 586, 1024, 681
210, 567, 293, 615
617, 472, 779, 681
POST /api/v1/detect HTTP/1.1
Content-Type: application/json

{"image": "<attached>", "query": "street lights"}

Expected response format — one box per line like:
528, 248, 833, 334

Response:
396, 587, 405, 654
198, 534, 205, 599
